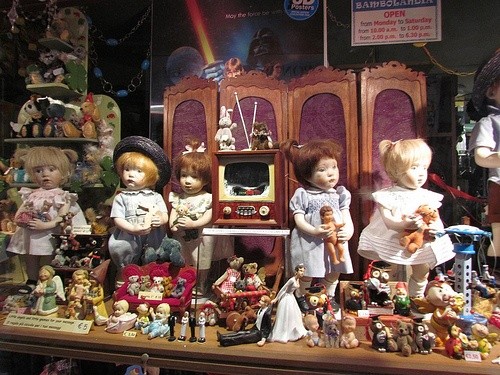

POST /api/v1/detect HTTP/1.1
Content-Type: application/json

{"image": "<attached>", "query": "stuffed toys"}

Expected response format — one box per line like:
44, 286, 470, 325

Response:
19, 42, 102, 139
388, 321, 416, 357
215, 254, 261, 298
16, 199, 52, 226
1, 146, 34, 233
400, 204, 437, 254
215, 105, 273, 151
77, 143, 115, 233
173, 199, 201, 243
127, 237, 189, 298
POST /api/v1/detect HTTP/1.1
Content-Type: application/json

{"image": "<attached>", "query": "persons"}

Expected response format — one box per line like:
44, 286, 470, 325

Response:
27, 259, 111, 329
214, 264, 359, 353
6, 147, 78, 296
106, 298, 207, 344
357, 139, 455, 313
279, 138, 354, 311
107, 135, 171, 268
167, 140, 214, 299
392, 263, 494, 360
466, 49, 500, 288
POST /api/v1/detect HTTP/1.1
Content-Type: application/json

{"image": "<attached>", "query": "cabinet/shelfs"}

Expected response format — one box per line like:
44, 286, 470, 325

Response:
0, 36, 110, 238
